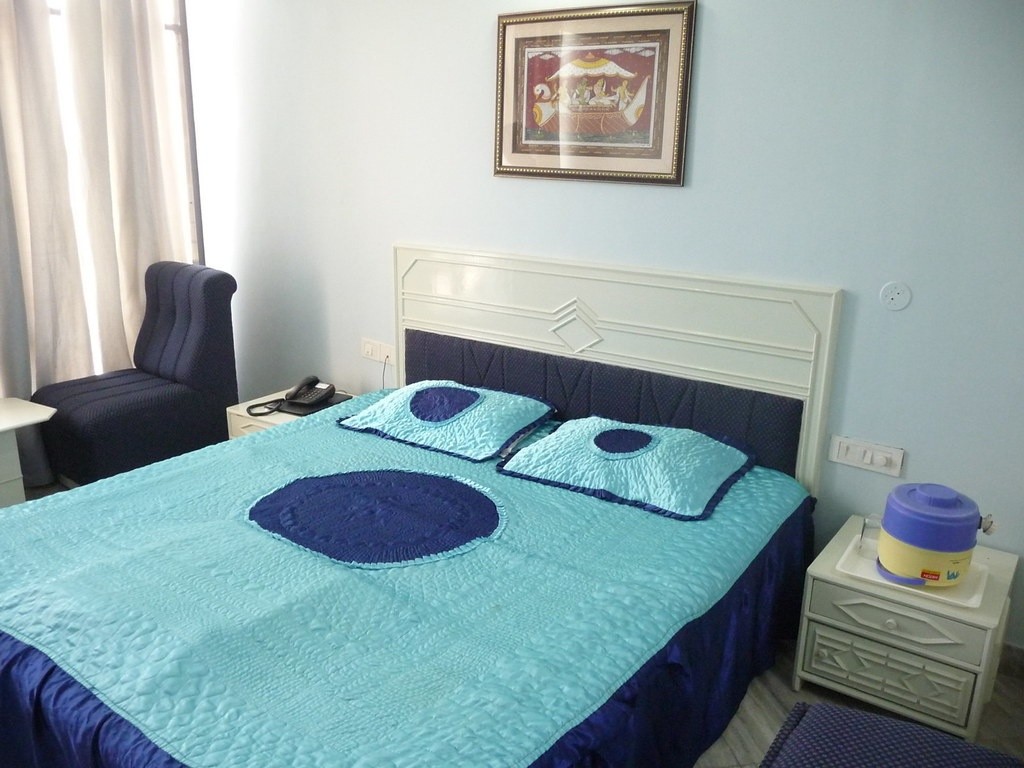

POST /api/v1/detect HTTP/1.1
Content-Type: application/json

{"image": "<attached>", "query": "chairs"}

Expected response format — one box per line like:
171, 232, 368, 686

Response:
32, 260, 238, 487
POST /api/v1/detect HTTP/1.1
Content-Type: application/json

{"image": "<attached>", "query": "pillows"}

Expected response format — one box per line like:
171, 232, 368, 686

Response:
337, 380, 557, 464
497, 412, 755, 522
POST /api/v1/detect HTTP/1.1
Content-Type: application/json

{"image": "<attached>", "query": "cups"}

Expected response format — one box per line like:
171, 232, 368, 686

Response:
859, 514, 880, 559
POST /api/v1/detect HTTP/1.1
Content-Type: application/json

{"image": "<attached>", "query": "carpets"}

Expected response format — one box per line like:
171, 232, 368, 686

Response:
759, 702, 1024, 768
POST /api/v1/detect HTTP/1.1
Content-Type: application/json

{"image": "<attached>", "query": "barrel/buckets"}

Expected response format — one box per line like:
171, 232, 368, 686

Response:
877, 483, 998, 588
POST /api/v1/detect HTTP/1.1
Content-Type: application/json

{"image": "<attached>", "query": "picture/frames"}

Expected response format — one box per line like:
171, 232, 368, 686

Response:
494, 0, 698, 187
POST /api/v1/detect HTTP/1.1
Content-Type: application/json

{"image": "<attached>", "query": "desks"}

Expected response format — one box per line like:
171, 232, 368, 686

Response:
0, 397, 57, 508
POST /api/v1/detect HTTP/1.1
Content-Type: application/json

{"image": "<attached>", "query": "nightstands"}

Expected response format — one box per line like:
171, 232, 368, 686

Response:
226, 386, 357, 440
792, 514, 1019, 742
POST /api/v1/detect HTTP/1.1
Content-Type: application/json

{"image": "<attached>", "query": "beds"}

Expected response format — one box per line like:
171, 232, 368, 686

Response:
0, 243, 842, 768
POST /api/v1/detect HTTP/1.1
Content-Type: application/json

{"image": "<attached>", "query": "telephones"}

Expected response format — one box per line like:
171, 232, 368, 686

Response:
284, 376, 335, 407
